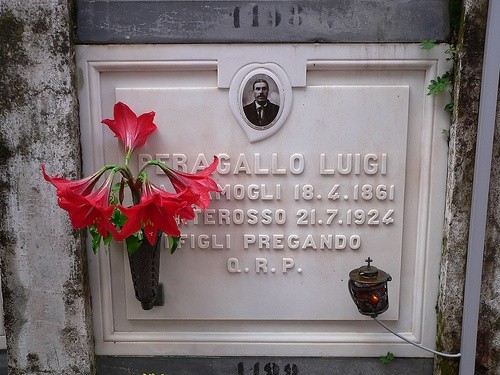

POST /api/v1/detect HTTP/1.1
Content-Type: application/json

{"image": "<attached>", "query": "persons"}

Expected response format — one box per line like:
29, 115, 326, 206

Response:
242, 77, 280, 127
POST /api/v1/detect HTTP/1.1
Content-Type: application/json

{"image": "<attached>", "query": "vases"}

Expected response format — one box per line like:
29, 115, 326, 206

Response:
122, 228, 166, 310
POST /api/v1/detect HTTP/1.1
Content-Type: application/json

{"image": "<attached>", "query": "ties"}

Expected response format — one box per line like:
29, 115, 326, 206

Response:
258, 107, 263, 124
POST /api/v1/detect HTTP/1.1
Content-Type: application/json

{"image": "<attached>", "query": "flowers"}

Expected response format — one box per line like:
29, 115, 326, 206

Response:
37, 102, 219, 255
